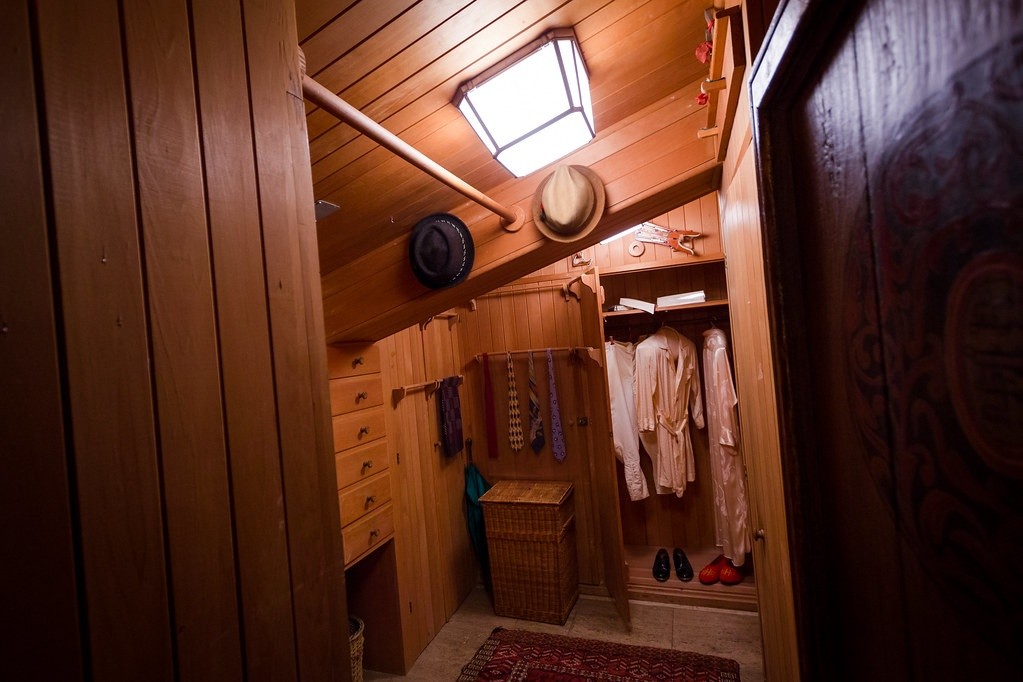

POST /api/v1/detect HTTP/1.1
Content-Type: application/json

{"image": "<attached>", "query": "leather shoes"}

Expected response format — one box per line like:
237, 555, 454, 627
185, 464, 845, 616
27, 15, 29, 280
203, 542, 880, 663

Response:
653, 548, 671, 581
673, 548, 694, 580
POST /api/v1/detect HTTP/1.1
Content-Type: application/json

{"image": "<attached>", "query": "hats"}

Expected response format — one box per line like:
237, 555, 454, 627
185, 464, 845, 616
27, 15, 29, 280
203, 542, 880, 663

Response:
408, 214, 475, 289
532, 165, 607, 244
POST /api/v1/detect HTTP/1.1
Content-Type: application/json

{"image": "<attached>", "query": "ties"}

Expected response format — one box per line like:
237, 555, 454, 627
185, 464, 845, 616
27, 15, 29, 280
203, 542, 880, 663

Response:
482, 352, 499, 461
506, 351, 524, 453
527, 349, 545, 454
546, 348, 566, 463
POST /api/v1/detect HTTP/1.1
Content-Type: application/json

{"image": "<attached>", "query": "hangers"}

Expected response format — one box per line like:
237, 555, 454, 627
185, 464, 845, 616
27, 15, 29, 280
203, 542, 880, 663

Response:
708, 314, 719, 330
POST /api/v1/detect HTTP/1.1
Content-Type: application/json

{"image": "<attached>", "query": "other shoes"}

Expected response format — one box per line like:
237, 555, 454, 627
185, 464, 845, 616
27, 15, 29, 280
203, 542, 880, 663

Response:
699, 554, 731, 584
719, 557, 751, 584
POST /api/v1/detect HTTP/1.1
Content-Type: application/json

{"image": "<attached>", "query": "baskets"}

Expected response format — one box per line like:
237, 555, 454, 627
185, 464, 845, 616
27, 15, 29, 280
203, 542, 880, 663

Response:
348, 614, 365, 682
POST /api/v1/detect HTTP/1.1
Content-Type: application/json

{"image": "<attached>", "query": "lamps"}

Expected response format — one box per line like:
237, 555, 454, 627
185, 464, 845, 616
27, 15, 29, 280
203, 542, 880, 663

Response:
452, 27, 596, 179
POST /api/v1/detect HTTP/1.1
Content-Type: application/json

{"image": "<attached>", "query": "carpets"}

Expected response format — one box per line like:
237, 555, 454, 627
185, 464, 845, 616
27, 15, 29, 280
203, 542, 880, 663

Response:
456, 626, 741, 682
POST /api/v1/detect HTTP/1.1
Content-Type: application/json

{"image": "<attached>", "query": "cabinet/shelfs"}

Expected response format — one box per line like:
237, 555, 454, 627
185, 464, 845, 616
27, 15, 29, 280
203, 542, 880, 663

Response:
327, 338, 395, 675
563, 249, 759, 632
